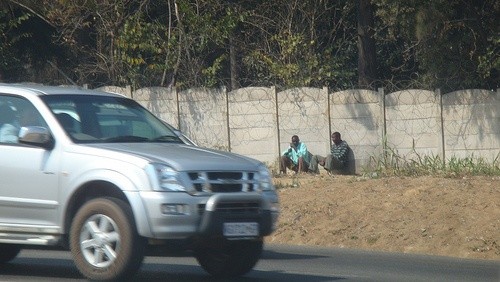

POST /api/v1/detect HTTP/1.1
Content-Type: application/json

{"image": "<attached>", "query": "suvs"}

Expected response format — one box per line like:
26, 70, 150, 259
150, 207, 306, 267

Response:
1, 82, 278, 282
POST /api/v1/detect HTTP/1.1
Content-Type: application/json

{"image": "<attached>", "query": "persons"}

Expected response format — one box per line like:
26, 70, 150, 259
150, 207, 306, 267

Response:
309, 131, 349, 175
0, 104, 38, 145
280, 135, 308, 176
